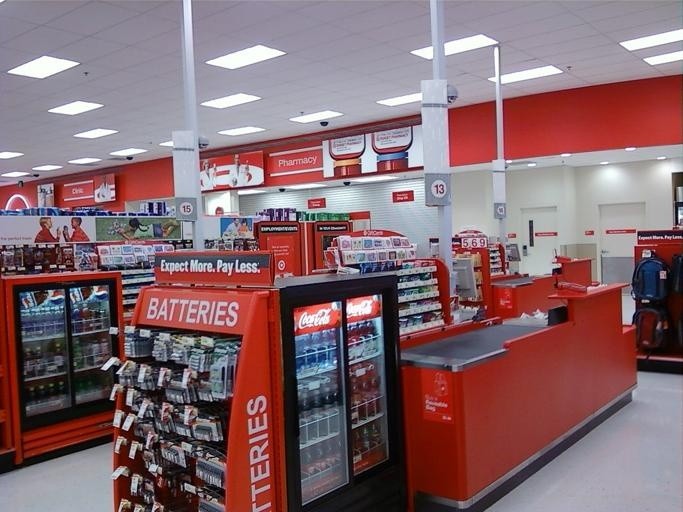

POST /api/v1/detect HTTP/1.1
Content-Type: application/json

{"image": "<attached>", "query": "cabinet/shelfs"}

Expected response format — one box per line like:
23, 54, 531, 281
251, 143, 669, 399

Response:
451, 241, 505, 311
341, 257, 446, 336
118, 269, 155, 336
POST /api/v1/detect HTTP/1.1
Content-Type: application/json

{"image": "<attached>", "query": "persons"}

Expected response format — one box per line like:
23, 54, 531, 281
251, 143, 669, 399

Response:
228, 153, 249, 186
99, 175, 112, 202
35, 215, 61, 242
119, 218, 180, 239
222, 218, 250, 237
63, 217, 89, 241
201, 159, 216, 189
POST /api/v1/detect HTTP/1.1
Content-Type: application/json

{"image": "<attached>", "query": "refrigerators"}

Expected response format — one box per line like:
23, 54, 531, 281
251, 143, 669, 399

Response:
279, 272, 408, 511
4, 271, 124, 465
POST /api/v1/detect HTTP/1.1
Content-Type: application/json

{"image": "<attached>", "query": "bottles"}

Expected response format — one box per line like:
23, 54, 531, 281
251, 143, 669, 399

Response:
300, 424, 384, 494
24, 336, 107, 377
28, 369, 109, 411
295, 319, 376, 374
297, 364, 381, 420
20, 304, 103, 339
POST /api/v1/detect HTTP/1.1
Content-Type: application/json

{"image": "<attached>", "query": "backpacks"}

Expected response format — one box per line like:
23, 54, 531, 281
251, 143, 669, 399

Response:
631, 251, 683, 364
